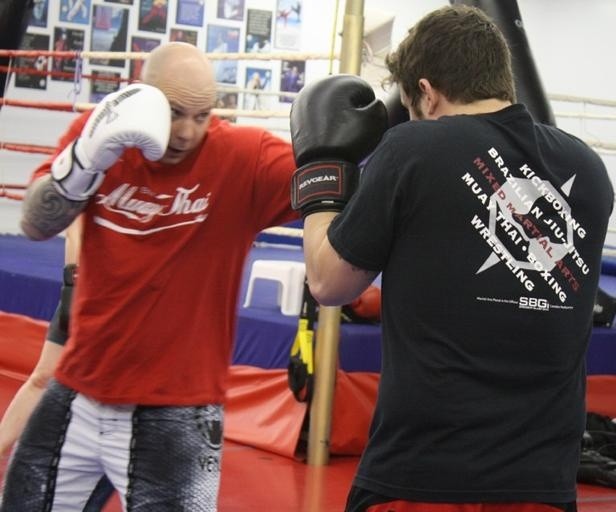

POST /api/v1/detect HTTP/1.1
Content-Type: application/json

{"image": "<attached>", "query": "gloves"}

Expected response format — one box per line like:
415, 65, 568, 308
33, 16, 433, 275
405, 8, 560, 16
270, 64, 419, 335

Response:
50, 83, 172, 202
288, 74, 410, 218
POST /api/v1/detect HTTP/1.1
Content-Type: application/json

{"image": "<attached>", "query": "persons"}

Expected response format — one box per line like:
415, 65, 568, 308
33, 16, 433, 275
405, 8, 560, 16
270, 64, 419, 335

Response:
0, 210, 82, 452
247, 70, 271, 110
0, 40, 408, 511
282, 65, 303, 103
290, 4, 614, 512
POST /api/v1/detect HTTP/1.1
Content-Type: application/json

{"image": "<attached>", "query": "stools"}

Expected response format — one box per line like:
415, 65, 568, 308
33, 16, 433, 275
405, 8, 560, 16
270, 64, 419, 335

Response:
242, 260, 306, 315
